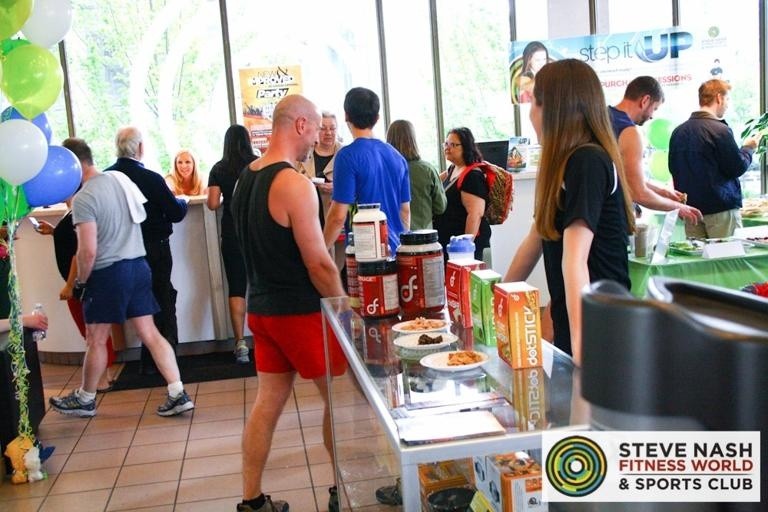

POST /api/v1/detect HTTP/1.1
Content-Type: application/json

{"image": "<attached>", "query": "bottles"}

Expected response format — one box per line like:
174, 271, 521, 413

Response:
363, 317, 401, 377
345, 232, 362, 312
352, 202, 389, 262
33, 302, 48, 341
349, 316, 366, 354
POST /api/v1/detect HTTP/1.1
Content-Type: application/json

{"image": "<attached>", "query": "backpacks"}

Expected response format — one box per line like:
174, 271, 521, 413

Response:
456, 160, 513, 225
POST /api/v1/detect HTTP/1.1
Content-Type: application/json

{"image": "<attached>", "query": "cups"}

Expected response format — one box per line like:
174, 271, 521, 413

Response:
633, 223, 650, 258
446, 233, 477, 261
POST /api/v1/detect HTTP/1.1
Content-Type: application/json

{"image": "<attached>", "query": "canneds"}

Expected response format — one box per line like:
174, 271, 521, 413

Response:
357, 259, 401, 319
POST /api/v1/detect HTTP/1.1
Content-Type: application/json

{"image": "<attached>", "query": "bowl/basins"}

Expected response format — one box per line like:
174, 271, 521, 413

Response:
425, 488, 476, 510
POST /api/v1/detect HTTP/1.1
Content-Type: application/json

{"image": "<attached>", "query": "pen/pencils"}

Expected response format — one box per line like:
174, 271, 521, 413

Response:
460, 407, 492, 413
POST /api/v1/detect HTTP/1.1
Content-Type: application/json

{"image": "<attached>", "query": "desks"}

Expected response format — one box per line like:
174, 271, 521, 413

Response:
653, 201, 768, 239
628, 224, 768, 299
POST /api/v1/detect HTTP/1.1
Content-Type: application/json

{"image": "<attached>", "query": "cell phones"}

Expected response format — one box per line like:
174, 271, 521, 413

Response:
28, 216, 43, 233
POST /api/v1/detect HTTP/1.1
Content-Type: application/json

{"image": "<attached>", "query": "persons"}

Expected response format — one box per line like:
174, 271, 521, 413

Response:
231, 95, 362, 511
0, 127, 207, 417
207, 124, 260, 364
710, 59, 722, 77
503, 58, 638, 367
312, 90, 513, 270
607, 76, 704, 226
668, 80, 761, 239
514, 42, 548, 103
508, 147, 521, 167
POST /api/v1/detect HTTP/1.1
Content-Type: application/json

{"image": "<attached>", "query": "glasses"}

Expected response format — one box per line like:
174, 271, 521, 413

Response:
441, 142, 462, 149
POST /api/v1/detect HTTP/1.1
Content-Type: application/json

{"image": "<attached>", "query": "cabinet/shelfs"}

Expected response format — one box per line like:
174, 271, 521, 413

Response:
319, 295, 592, 512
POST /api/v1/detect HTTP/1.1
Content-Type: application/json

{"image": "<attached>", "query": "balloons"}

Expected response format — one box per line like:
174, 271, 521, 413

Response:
0, 2, 82, 225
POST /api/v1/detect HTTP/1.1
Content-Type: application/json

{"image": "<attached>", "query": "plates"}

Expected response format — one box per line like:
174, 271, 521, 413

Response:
391, 319, 450, 334
393, 331, 460, 350
419, 350, 490, 373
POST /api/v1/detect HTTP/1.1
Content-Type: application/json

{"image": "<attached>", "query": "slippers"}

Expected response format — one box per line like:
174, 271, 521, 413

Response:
97, 379, 116, 394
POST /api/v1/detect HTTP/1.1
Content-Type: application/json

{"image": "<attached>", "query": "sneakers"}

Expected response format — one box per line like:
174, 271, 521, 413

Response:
156, 389, 195, 416
232, 340, 249, 364
237, 494, 289, 511
375, 478, 402, 505
48, 388, 96, 418
328, 488, 340, 511
